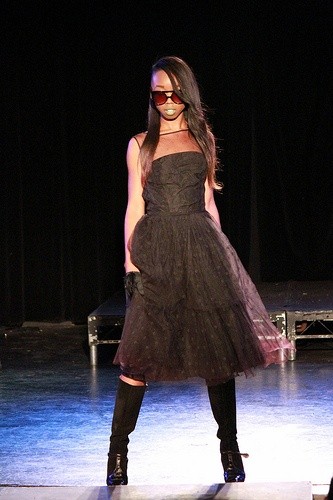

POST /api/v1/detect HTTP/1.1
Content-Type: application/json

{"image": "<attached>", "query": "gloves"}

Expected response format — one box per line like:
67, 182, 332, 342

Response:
125, 271, 144, 308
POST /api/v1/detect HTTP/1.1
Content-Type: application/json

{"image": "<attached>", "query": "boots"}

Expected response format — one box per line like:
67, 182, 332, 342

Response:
205, 376, 248, 483
106, 378, 148, 485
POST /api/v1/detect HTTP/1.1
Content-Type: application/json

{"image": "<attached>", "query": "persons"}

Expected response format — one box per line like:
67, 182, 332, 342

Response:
105, 57, 296, 487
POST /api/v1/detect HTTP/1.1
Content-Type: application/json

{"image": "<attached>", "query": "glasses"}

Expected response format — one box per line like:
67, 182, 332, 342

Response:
151, 90, 184, 105
